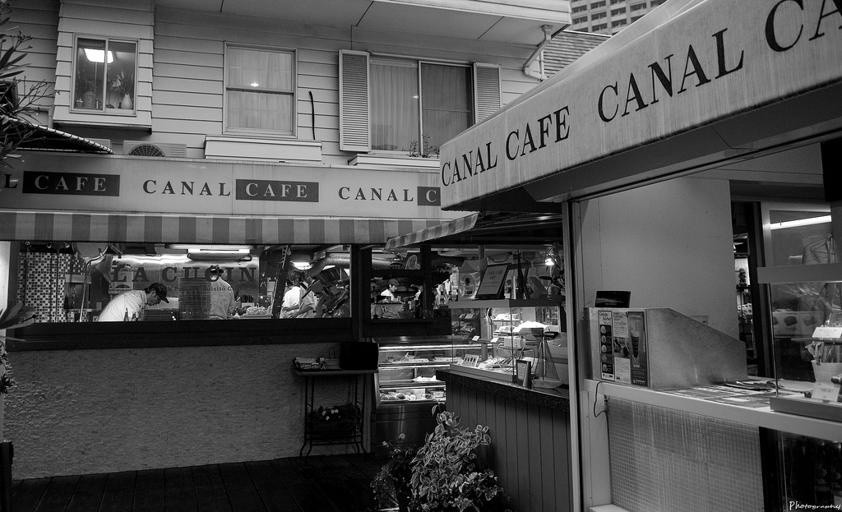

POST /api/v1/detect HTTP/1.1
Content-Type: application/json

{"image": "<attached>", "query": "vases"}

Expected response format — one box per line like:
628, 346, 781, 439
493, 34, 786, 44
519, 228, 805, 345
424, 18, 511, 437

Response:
393, 468, 412, 512
121, 93, 133, 109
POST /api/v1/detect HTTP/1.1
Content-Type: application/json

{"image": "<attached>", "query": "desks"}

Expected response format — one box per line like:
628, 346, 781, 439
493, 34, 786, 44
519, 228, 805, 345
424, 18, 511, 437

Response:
292, 361, 378, 463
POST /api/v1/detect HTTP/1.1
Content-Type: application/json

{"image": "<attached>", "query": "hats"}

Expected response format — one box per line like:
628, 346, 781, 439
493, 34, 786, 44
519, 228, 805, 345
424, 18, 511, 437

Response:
149, 282, 170, 304
387, 279, 400, 286
210, 265, 224, 274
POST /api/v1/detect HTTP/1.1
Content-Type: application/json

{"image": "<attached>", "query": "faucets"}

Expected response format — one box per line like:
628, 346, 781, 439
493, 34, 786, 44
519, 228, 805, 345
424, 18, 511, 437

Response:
516, 358, 532, 388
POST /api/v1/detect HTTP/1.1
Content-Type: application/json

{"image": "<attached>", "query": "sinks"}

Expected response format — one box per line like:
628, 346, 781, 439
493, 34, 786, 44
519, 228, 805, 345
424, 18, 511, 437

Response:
532, 378, 563, 390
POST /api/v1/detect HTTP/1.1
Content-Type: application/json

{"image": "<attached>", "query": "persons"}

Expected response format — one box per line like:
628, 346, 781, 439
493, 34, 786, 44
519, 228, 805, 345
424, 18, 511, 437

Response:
281, 270, 316, 317
98, 282, 170, 322
280, 281, 301, 319
200, 265, 242, 319
378, 279, 402, 302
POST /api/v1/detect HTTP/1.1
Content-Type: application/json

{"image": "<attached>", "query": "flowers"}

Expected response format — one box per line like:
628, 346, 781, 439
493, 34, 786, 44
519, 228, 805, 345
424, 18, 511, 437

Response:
111, 69, 134, 95
370, 431, 418, 510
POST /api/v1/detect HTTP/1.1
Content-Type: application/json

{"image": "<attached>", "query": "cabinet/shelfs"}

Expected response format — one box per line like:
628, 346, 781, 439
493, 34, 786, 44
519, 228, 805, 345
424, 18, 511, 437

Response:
379, 342, 493, 405
